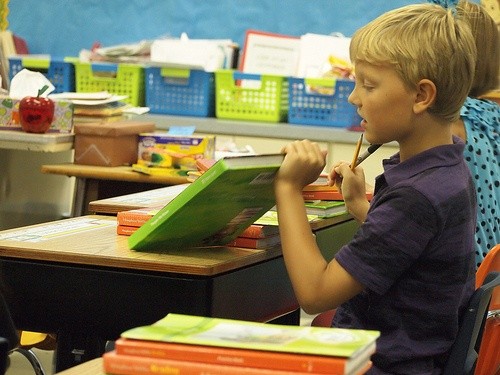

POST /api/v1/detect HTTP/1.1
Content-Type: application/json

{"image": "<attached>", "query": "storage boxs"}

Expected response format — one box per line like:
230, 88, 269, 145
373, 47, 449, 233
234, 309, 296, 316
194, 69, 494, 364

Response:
7, 55, 362, 169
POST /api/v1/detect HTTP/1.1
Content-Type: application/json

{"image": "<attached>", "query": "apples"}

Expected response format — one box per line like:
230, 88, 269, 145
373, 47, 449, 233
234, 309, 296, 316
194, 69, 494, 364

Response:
19, 86, 55, 133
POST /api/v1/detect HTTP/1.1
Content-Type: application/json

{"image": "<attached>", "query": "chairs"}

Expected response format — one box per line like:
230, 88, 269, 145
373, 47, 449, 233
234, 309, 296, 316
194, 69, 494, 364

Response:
441, 245, 500, 375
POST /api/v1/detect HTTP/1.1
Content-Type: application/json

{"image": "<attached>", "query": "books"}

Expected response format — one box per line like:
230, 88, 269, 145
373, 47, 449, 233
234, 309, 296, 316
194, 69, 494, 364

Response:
103, 313, 381, 375
117, 153, 374, 252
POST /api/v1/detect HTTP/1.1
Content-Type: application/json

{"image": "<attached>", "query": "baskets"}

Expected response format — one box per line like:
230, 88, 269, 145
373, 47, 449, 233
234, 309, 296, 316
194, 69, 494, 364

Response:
64, 58, 145, 106
7, 55, 75, 94
286, 76, 363, 127
145, 67, 215, 118
214, 69, 288, 123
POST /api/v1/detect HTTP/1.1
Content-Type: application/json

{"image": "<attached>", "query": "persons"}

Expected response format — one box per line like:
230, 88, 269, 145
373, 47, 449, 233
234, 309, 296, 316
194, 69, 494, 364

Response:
274, 0, 477, 375
457, 0, 500, 273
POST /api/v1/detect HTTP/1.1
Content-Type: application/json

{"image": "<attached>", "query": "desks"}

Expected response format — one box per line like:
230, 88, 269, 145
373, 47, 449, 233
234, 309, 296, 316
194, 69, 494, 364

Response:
0, 92, 372, 375
